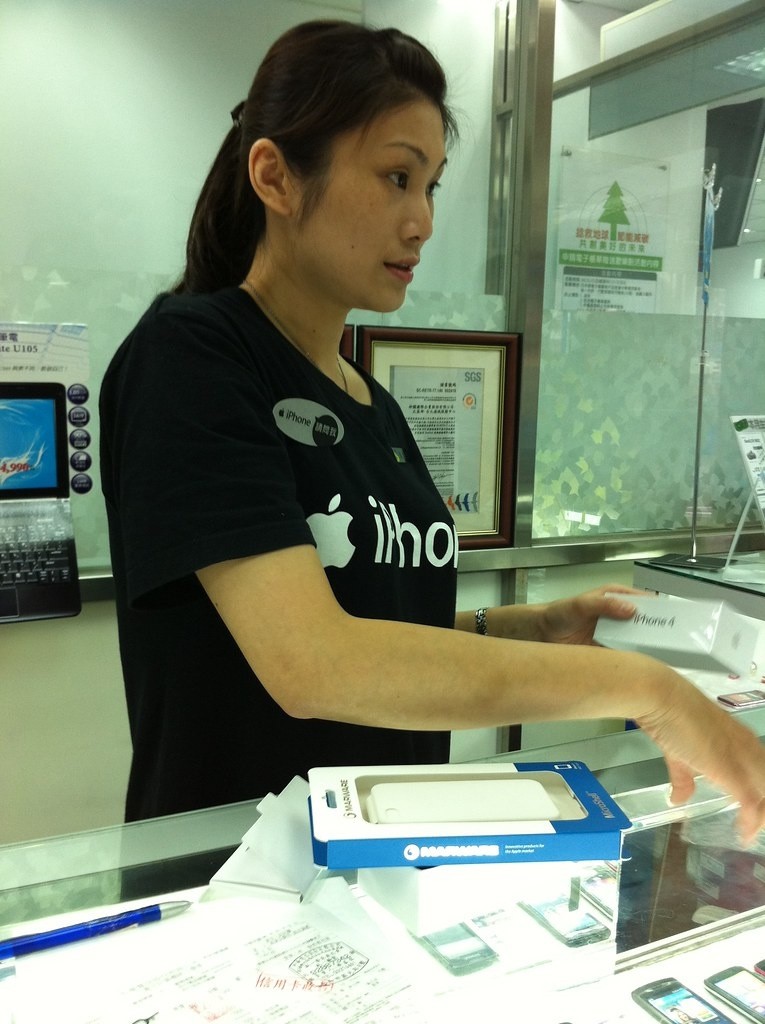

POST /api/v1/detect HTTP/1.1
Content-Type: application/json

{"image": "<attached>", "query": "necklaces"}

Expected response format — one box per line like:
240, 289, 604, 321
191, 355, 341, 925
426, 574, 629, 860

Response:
243, 279, 348, 396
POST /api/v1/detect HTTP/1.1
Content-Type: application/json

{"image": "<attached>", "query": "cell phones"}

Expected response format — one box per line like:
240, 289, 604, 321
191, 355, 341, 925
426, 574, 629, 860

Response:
407, 912, 496, 977
367, 779, 560, 823
517, 864, 619, 949
632, 977, 737, 1024
704, 966, 765, 1024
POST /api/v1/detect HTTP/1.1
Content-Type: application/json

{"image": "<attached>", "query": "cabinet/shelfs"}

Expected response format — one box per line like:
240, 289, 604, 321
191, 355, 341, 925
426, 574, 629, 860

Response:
2, 701, 765, 1024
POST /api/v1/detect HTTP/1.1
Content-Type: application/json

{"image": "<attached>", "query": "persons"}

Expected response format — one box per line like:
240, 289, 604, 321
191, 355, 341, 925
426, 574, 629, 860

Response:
96, 20, 765, 902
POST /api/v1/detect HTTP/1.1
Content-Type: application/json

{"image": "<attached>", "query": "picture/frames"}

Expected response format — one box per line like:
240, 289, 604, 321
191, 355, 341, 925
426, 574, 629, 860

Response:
352, 320, 522, 553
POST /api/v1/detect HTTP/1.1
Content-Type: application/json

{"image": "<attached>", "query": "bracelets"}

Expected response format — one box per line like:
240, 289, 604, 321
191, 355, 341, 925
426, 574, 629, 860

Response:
474, 607, 487, 636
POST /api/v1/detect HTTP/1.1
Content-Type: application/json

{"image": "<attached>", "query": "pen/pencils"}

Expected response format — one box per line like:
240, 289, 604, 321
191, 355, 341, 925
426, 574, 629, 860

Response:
2, 898, 195, 964
